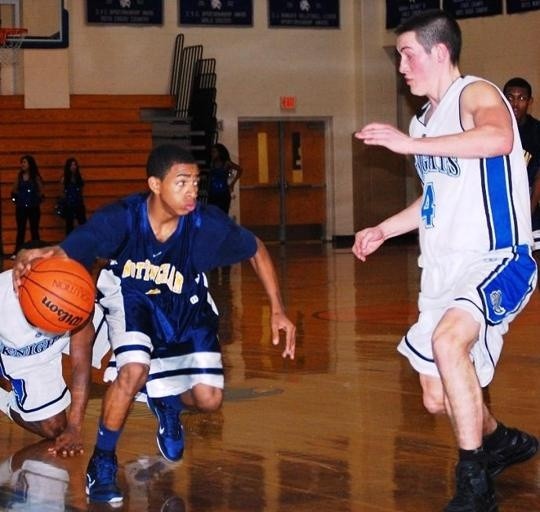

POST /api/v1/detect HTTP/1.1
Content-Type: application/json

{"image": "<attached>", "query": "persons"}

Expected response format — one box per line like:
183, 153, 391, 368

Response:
502, 77, 540, 285
1, 438, 86, 512
1, 239, 95, 488
54, 157, 87, 237
206, 143, 243, 275
11, 144, 298, 504
116, 455, 187, 512
11, 155, 45, 259
351, 10, 539, 511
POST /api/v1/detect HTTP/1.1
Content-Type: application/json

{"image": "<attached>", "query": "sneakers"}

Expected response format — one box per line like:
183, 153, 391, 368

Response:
146, 393, 184, 462
485, 428, 540, 478
443, 464, 497, 512
84, 451, 123, 503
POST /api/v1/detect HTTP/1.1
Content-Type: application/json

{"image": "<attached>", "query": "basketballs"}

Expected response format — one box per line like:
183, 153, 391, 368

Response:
20, 257, 95, 334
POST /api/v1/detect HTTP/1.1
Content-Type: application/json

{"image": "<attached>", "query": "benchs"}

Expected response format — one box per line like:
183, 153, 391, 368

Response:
1, 95, 175, 253
